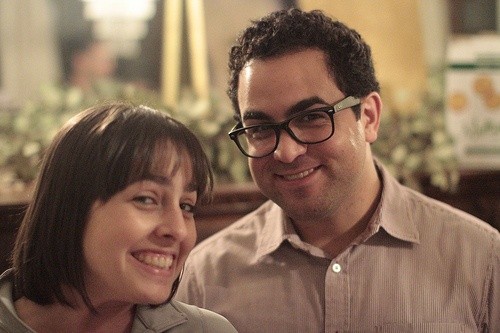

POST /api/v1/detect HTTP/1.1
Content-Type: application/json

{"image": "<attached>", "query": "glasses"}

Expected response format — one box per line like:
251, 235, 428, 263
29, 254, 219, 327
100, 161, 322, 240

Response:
228, 96, 363, 158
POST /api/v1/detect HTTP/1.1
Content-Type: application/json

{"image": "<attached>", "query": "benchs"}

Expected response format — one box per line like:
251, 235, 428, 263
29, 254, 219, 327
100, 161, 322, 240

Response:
0, 166, 500, 273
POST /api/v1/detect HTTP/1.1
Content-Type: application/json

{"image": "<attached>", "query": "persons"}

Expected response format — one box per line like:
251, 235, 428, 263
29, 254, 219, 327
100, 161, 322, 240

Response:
0, 102, 238, 333
174, 8, 500, 333
